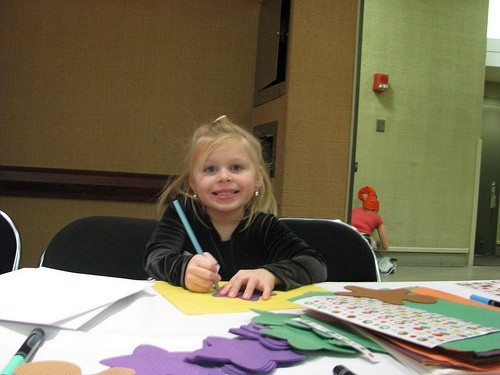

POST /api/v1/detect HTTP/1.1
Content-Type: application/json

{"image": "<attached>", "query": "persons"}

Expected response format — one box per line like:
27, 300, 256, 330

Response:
142, 114, 328, 300
351, 186, 388, 250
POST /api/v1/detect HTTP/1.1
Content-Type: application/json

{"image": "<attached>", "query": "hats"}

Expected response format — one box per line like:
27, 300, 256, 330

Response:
364, 196, 379, 211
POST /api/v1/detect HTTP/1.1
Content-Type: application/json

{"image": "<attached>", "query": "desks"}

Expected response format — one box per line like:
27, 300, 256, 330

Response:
0, 280, 415, 375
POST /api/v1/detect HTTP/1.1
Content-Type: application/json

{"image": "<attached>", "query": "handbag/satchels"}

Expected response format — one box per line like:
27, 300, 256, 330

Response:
378, 257, 394, 275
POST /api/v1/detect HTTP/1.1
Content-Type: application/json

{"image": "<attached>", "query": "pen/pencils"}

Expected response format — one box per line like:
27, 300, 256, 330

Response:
173, 199, 222, 291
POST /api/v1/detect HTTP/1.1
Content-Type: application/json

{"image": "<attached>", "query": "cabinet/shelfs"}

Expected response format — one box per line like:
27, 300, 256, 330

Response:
253, 0, 357, 108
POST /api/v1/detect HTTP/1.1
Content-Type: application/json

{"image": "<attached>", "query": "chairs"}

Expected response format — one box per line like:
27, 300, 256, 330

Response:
280, 217, 381, 284
39, 216, 158, 280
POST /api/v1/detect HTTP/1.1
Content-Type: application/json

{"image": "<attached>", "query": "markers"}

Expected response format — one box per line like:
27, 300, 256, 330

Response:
470, 294, 500, 309
1, 327, 46, 375
333, 364, 357, 375
